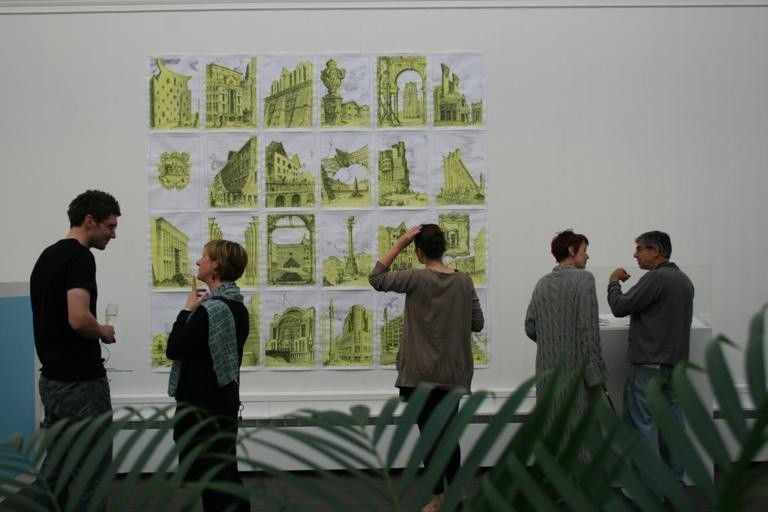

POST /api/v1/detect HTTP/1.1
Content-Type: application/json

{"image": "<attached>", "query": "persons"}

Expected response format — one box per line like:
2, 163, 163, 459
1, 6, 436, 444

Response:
607, 229, 697, 502
29, 188, 124, 510
367, 224, 486, 510
165, 239, 252, 510
524, 228, 611, 459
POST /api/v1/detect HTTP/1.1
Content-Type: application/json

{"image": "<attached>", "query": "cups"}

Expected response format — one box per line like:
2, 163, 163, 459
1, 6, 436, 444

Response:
104, 304, 118, 326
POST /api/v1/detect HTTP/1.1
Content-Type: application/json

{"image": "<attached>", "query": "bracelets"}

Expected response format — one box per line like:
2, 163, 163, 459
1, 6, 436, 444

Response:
183, 308, 192, 311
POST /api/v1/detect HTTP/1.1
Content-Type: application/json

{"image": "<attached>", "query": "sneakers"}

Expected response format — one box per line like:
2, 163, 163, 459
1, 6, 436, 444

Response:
421, 495, 469, 511
